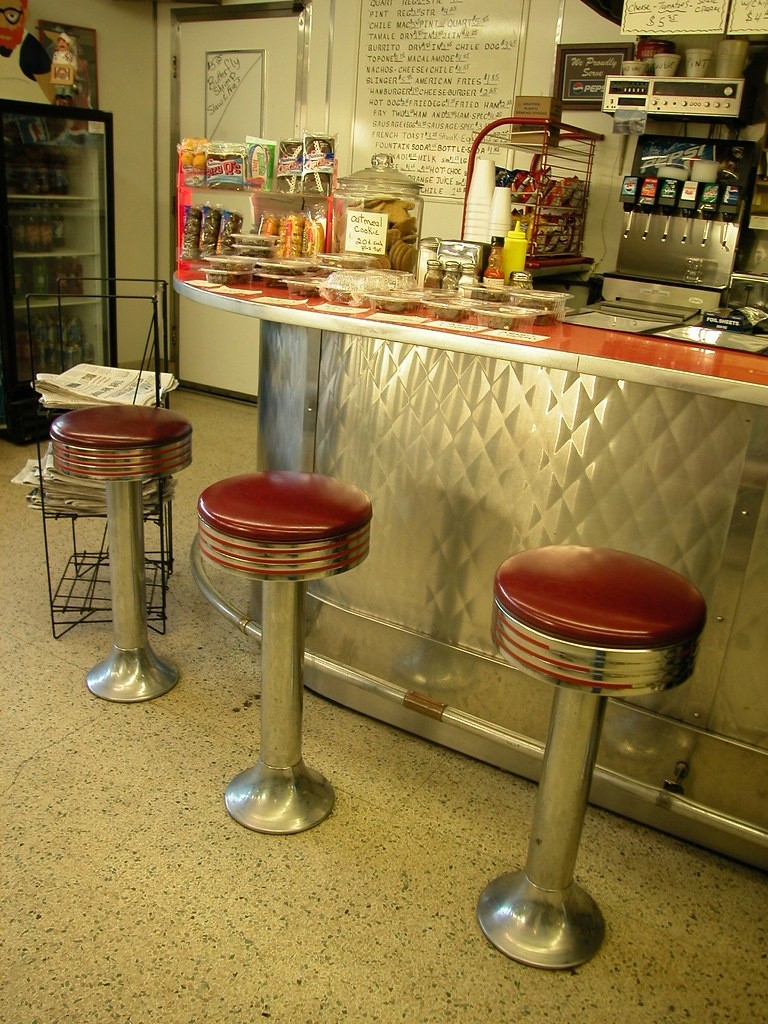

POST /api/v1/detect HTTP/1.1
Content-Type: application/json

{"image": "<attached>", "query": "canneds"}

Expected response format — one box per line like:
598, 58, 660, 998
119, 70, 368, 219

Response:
15, 314, 93, 375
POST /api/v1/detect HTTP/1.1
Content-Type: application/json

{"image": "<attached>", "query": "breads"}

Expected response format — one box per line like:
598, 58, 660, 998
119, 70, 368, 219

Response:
261, 214, 324, 258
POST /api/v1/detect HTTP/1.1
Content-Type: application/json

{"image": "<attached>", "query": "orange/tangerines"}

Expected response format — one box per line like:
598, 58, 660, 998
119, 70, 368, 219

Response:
180, 151, 207, 169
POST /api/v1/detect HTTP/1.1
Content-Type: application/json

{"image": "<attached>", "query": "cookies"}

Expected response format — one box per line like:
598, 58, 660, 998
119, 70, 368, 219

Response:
333, 195, 419, 273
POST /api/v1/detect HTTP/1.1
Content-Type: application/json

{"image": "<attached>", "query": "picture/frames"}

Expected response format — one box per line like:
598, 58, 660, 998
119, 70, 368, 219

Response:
554, 42, 635, 112
37, 19, 99, 109
49, 63, 74, 85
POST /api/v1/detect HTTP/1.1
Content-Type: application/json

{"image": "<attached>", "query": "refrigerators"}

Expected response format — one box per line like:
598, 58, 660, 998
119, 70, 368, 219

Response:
0, 99, 116, 442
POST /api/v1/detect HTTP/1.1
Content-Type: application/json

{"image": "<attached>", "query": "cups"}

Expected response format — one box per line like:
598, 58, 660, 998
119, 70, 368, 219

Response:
623, 61, 647, 76
657, 163, 690, 180
654, 54, 682, 77
462, 159, 510, 245
716, 40, 747, 78
691, 160, 719, 182
685, 49, 713, 76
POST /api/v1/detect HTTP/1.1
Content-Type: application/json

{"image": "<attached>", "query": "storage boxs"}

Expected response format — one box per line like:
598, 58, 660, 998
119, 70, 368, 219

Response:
510, 93, 564, 148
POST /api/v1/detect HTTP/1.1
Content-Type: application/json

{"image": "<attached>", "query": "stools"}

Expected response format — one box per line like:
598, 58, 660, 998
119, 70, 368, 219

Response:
196, 470, 371, 835
53, 408, 193, 708
476, 548, 705, 968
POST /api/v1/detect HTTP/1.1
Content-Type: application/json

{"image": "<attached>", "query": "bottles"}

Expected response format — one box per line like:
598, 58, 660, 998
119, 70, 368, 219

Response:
423, 260, 442, 288
510, 271, 533, 291
459, 264, 478, 285
440, 261, 460, 289
504, 220, 528, 285
4, 152, 93, 372
483, 235, 505, 285
